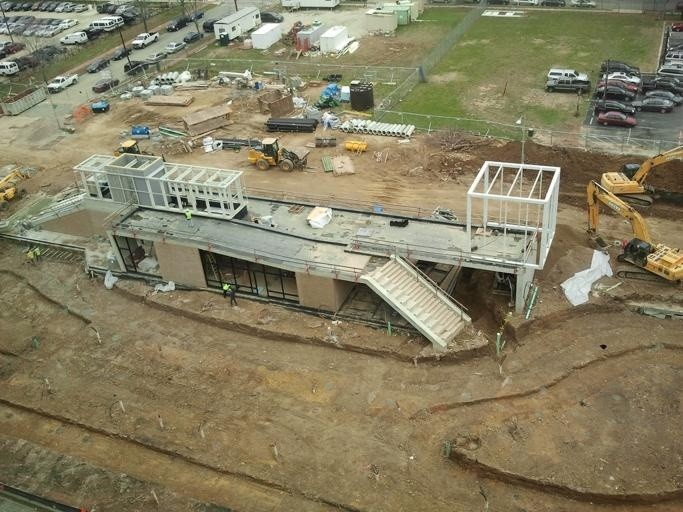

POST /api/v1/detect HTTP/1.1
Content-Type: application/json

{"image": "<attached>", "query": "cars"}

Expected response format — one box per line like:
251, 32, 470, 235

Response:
0, 0, 218, 93
486, 0, 596, 8
260, 11, 284, 23
544, 13, 683, 126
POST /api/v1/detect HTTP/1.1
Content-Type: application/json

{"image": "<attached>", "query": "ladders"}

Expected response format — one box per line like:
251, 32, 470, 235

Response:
207, 253, 224, 282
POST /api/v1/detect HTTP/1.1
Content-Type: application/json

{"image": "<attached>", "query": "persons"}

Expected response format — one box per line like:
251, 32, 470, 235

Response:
221, 284, 230, 298
24, 250, 35, 264
228, 288, 237, 307
31, 246, 41, 262
183, 208, 193, 228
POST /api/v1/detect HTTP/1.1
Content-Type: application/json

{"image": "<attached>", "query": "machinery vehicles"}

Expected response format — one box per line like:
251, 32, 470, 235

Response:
586, 146, 683, 280
114, 140, 153, 155
0, 168, 32, 212
247, 138, 310, 171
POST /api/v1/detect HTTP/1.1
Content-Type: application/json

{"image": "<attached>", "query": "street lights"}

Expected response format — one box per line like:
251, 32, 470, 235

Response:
516, 114, 534, 163
